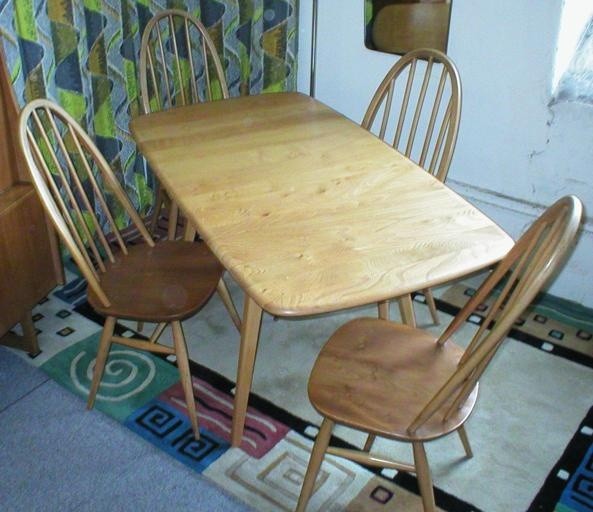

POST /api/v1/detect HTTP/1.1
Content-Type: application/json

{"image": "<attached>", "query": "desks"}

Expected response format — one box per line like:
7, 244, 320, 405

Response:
128, 90, 516, 448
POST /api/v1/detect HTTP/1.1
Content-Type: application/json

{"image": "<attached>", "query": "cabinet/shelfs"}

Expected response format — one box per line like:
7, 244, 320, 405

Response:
1, 52, 67, 359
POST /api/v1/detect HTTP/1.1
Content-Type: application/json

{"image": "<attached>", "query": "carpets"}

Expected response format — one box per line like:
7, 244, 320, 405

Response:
1, 238, 593, 510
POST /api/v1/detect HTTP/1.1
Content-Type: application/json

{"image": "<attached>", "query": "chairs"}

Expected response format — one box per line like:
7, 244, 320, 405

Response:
138, 9, 229, 242
295, 194, 583, 511
360, 46, 462, 326
19, 97, 241, 442
